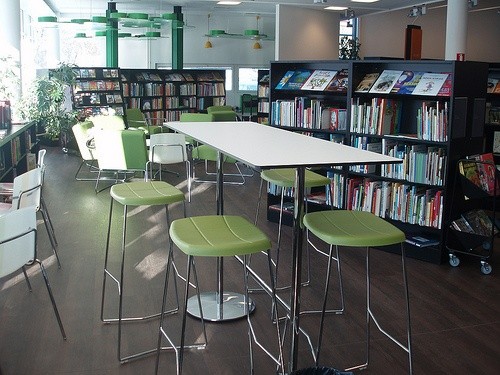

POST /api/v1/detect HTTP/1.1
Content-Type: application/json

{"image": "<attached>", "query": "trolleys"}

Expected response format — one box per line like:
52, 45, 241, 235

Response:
442, 153, 500, 275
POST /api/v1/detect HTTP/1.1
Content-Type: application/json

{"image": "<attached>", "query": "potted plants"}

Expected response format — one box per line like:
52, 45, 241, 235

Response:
18, 62, 80, 154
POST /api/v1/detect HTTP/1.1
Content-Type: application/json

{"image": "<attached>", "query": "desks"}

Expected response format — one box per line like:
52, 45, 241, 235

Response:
162, 121, 403, 375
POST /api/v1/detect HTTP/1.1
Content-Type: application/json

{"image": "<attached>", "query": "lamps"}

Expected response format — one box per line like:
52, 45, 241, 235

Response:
38, 0, 195, 40
252, 16, 263, 49
200, 29, 267, 40
204, 14, 212, 48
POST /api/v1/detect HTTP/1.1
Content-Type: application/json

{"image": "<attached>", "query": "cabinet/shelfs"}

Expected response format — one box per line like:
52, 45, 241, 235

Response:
257, 56, 500, 265
48, 67, 226, 157
0, 120, 41, 204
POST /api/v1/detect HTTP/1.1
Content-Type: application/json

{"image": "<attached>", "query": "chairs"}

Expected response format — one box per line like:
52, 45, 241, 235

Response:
0, 149, 71, 340
72, 106, 254, 203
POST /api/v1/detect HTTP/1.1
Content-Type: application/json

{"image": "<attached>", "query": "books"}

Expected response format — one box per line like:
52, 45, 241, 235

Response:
268, 70, 500, 249
72, 69, 268, 135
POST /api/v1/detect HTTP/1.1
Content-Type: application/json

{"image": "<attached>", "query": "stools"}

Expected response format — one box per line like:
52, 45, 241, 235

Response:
101, 167, 413, 375
